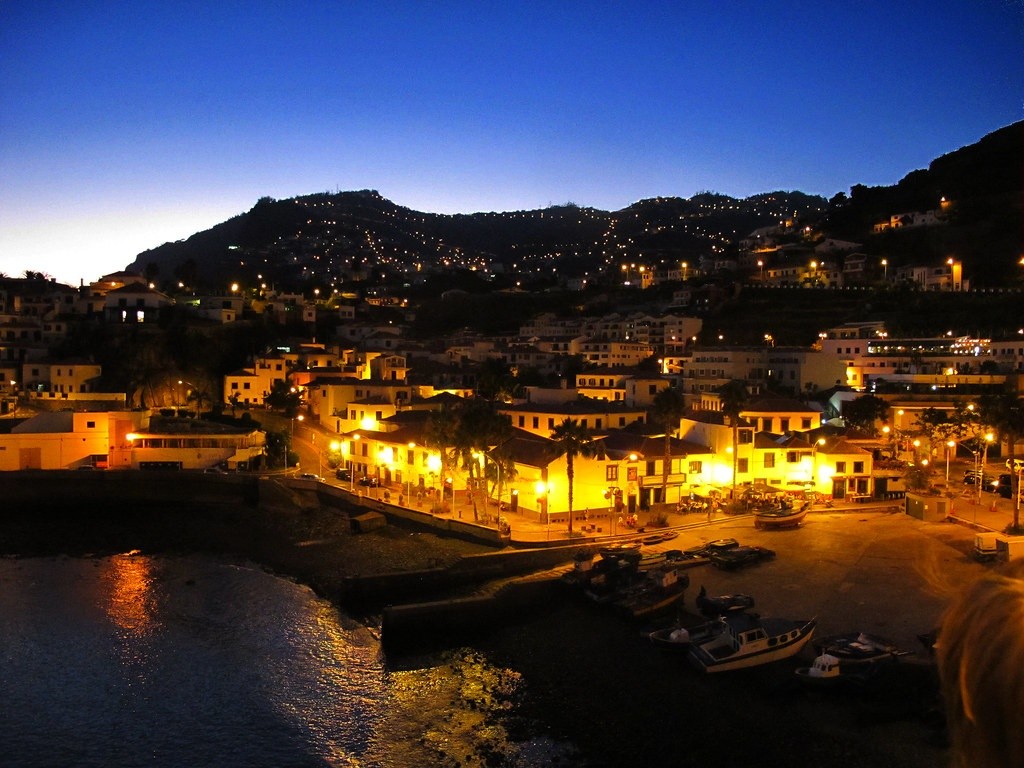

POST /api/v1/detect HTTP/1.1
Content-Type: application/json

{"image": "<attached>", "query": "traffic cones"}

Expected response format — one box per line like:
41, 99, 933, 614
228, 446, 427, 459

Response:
989, 500, 998, 512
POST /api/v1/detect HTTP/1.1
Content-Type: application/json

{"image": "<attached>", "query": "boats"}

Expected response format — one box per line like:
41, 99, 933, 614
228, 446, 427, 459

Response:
811, 632, 860, 658
617, 566, 689, 620
696, 595, 756, 617
663, 530, 681, 542
598, 538, 774, 572
792, 634, 900, 690
749, 496, 812, 530
645, 614, 760, 651
643, 536, 666, 546
686, 614, 820, 676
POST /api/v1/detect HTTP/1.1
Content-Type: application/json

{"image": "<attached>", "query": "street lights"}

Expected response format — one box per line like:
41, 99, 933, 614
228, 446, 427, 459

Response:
983, 434, 993, 466
177, 380, 201, 421
944, 440, 954, 489
430, 457, 440, 515
290, 416, 304, 452
472, 451, 502, 530
614, 454, 637, 518
811, 439, 826, 501
535, 481, 555, 540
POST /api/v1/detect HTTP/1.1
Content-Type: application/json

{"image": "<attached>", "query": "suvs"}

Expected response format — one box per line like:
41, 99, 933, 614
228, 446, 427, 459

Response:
301, 473, 326, 483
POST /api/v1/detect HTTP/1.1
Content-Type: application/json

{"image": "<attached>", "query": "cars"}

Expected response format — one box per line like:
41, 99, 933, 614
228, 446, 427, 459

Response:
336, 468, 355, 482
962, 469, 1019, 499
1004, 458, 1024, 473
359, 476, 381, 487
